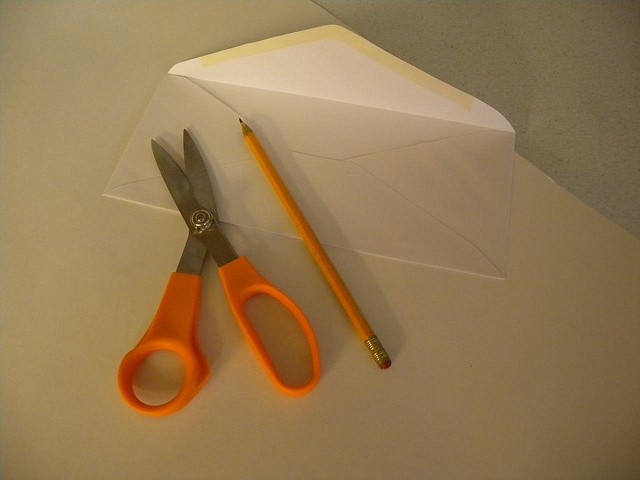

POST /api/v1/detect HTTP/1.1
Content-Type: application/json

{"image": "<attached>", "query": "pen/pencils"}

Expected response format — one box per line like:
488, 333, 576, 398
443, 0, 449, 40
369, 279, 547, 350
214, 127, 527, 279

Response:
237, 118, 392, 371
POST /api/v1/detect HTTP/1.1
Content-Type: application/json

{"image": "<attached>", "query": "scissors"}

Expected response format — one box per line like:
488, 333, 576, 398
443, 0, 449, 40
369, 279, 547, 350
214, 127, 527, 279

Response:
117, 129, 321, 419
